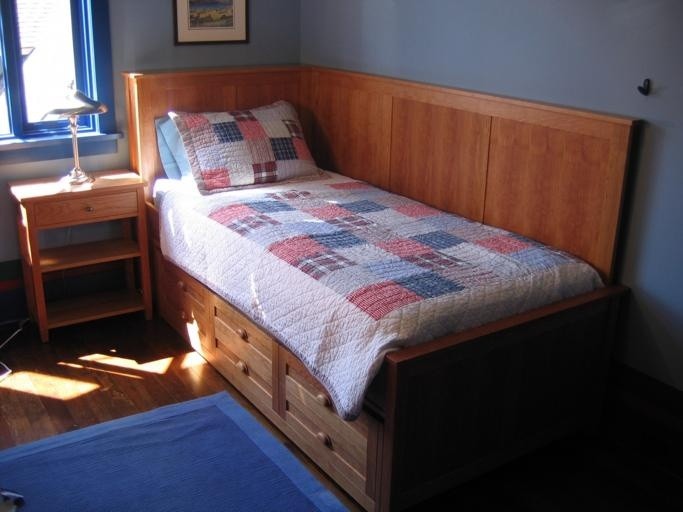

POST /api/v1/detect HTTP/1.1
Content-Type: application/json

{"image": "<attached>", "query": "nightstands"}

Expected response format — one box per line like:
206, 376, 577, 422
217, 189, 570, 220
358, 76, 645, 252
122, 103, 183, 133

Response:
8, 170, 155, 341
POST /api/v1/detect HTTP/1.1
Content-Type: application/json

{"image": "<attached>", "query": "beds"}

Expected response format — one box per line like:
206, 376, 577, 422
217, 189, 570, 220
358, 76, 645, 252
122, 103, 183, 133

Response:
122, 61, 641, 511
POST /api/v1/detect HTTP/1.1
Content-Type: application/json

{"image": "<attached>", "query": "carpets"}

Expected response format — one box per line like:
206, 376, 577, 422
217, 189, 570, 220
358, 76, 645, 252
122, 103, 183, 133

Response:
0, 388, 354, 512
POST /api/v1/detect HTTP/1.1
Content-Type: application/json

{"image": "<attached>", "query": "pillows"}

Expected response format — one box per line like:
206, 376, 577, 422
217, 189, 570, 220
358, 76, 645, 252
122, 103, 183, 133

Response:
153, 100, 320, 192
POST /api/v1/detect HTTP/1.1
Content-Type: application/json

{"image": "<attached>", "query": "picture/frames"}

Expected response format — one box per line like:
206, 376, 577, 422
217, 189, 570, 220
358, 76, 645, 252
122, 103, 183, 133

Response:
172, 1, 249, 46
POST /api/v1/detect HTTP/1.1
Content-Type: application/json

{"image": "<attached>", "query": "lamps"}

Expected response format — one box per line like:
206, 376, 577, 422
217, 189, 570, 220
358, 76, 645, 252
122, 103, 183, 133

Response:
43, 79, 108, 187
